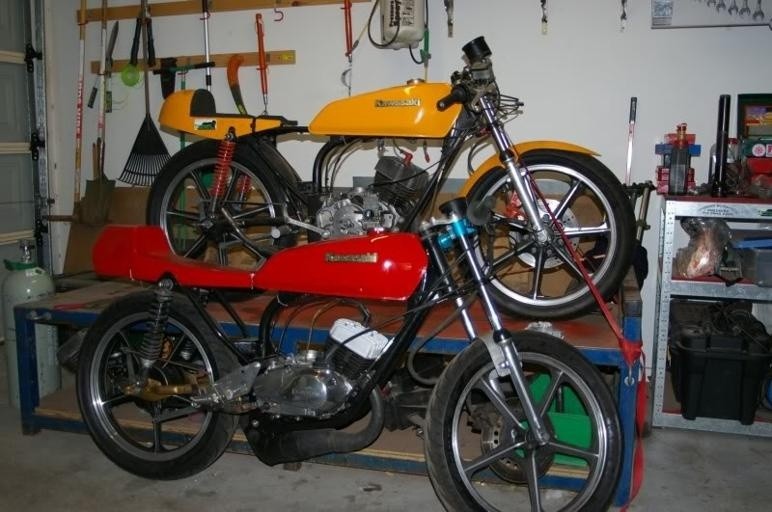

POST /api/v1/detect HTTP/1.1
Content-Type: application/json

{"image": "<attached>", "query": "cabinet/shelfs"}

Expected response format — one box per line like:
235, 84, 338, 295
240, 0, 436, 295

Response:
13, 278, 643, 506
651, 195, 771, 438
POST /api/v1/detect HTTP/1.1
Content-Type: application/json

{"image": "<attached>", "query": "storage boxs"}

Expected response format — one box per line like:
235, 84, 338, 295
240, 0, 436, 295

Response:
730, 229, 772, 287
667, 297, 770, 425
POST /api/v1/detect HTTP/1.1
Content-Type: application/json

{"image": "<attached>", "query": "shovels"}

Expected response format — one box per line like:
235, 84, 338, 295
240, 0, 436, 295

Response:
78, 0, 116, 228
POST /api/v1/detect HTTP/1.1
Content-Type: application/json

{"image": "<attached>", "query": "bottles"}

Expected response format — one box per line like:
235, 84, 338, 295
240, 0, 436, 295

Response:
669, 124, 688, 195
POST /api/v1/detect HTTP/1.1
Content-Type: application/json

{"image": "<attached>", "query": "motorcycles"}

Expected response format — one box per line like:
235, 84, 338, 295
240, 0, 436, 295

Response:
148, 35, 638, 319
77, 195, 624, 512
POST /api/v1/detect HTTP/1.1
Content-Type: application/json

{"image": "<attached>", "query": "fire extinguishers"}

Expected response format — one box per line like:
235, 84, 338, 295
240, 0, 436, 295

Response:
4, 240, 61, 411
